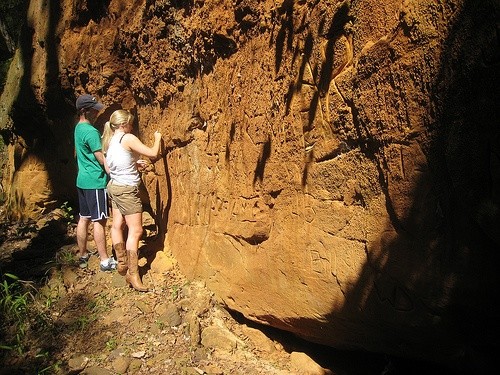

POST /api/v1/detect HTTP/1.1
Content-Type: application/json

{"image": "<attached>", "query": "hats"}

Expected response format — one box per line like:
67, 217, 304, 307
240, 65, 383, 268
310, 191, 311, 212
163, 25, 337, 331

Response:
76, 94, 104, 111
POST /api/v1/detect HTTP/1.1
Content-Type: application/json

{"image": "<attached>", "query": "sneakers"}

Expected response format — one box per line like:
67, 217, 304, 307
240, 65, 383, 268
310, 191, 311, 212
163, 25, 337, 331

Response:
78, 253, 93, 268
99, 257, 119, 271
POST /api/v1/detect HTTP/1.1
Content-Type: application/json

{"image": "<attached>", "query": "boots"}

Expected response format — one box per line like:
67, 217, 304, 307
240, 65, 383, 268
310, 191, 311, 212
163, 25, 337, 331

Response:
125, 249, 148, 292
113, 242, 128, 275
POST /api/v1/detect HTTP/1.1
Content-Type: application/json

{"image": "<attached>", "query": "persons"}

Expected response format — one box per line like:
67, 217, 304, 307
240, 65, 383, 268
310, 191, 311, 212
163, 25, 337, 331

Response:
74, 95, 120, 272
100, 108, 162, 293
